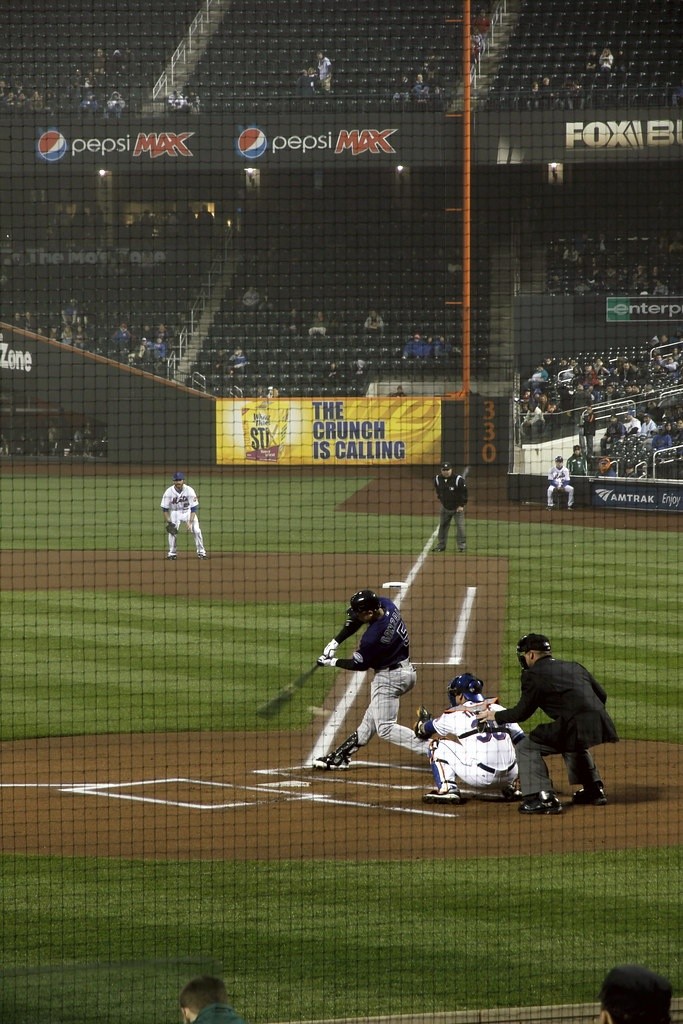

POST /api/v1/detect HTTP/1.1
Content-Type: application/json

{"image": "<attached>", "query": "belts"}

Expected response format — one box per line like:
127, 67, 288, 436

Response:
478, 762, 518, 774
390, 663, 401, 671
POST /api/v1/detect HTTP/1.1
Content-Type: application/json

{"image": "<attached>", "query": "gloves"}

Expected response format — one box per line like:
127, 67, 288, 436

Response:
323, 639, 339, 659
317, 658, 338, 668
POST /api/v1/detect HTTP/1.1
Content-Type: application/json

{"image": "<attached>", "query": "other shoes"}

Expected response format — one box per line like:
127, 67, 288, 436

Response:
432, 547, 444, 552
168, 554, 178, 561
546, 506, 554, 510
198, 553, 207, 559
568, 505, 575, 510
459, 547, 462, 552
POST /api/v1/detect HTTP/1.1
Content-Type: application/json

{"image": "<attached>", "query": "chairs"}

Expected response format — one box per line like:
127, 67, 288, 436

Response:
0, 1, 683, 474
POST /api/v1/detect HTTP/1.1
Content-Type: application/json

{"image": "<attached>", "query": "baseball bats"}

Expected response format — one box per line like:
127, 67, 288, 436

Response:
255, 656, 330, 720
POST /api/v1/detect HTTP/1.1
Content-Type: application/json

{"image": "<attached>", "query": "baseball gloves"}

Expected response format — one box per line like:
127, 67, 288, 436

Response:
165, 521, 179, 538
413, 703, 435, 743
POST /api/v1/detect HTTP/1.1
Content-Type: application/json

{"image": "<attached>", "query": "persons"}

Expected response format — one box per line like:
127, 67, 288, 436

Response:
519, 330, 683, 478
476, 633, 619, 815
160, 472, 208, 560
413, 673, 526, 804
312, 590, 435, 772
0, 299, 178, 457
77, 48, 126, 118
597, 964, 674, 1024
528, 48, 614, 109
666, 73, 683, 106
179, 976, 249, 1024
470, 9, 491, 83
0, 79, 55, 114
546, 456, 575, 512
548, 228, 683, 296
431, 462, 469, 552
390, 62, 445, 104
214, 309, 450, 398
167, 91, 200, 113
297, 52, 333, 95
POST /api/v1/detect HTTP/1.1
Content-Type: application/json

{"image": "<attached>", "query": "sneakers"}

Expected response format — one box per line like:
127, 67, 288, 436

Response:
519, 793, 563, 815
422, 786, 460, 804
312, 752, 350, 770
573, 787, 606, 805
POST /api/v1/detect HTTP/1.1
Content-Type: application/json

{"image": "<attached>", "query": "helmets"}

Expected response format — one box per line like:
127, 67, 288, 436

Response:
453, 674, 484, 702
556, 457, 562, 462
351, 589, 381, 613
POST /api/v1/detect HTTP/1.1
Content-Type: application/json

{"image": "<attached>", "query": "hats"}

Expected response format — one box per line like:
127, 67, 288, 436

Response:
441, 462, 452, 470
172, 473, 185, 482
517, 633, 552, 652
